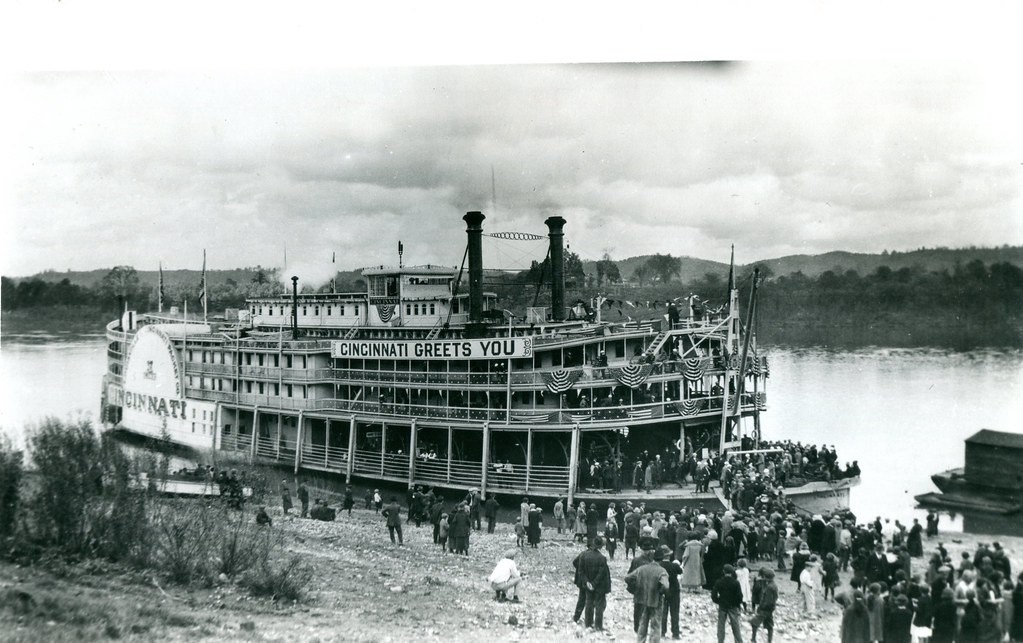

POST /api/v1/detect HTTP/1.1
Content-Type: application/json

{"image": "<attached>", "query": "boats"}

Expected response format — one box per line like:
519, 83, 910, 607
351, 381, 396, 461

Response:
103, 468, 253, 502
915, 428, 1023, 514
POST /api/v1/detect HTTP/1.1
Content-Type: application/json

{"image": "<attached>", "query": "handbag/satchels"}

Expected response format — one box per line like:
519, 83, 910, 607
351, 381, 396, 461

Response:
748, 614, 762, 627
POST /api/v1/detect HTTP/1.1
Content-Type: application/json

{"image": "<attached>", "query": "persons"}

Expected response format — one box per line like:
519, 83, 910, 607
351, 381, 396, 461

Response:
65, 295, 1023, 642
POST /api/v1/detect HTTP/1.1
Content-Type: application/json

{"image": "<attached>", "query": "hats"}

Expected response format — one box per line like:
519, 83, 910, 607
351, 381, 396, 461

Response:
654, 549, 664, 559
661, 544, 674, 555
641, 540, 654, 549
505, 549, 517, 557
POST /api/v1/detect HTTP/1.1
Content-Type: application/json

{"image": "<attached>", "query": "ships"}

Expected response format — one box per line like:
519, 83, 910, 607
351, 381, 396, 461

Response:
101, 210, 861, 528
